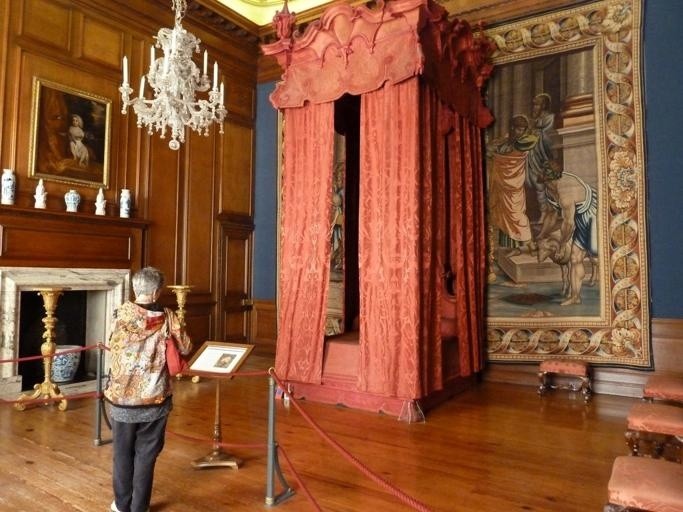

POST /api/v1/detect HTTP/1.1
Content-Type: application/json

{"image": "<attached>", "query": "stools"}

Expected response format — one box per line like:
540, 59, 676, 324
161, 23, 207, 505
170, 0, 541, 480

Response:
642, 375, 683, 402
604, 454, 683, 512
624, 403, 683, 457
536, 360, 594, 404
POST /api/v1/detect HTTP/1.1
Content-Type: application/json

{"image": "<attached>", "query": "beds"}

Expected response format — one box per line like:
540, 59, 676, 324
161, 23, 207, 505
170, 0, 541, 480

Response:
258, 0, 495, 423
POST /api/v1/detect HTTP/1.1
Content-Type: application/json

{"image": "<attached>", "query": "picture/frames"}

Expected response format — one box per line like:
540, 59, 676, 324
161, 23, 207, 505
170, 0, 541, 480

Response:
182, 341, 255, 380
26, 75, 112, 192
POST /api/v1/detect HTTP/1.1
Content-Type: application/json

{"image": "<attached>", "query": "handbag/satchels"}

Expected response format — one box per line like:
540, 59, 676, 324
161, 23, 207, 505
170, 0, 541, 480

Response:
165, 335, 188, 377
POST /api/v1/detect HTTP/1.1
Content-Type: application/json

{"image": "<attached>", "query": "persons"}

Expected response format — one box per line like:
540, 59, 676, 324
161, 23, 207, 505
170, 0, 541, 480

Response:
104, 267, 194, 511
329, 161, 343, 271
69, 114, 88, 168
489, 93, 598, 306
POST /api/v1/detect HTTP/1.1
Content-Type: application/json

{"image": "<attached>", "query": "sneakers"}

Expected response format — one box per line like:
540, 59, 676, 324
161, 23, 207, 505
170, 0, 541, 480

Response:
110, 500, 150, 512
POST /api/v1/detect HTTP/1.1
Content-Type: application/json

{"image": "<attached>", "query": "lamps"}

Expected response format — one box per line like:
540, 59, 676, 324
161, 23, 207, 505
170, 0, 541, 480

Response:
117, 0, 229, 151
